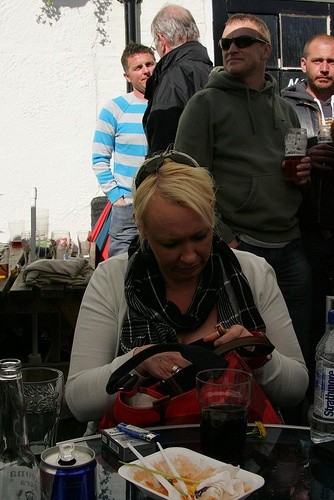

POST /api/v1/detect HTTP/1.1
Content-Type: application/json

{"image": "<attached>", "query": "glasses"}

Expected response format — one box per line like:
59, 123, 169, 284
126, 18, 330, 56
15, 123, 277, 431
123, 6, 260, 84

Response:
136, 153, 196, 188
219, 34, 268, 50
149, 32, 162, 51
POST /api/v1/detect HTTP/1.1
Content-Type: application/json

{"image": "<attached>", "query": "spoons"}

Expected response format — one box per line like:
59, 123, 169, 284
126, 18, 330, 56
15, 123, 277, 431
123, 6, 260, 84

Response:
127, 442, 182, 500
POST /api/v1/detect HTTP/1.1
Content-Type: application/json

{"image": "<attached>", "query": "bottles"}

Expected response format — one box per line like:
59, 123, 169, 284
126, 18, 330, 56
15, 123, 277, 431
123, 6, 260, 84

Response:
310, 309, 334, 444
0, 358, 40, 470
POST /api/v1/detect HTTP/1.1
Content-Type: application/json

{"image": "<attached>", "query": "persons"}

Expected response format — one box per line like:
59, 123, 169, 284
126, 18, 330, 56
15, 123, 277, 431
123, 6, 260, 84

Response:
173, 14, 310, 362
65, 152, 309, 438
278, 34, 334, 402
142, 4, 214, 160
91, 45, 157, 258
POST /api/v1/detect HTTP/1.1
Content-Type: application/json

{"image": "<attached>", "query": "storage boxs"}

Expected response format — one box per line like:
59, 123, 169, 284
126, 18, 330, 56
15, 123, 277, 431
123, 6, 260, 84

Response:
101, 428, 156, 460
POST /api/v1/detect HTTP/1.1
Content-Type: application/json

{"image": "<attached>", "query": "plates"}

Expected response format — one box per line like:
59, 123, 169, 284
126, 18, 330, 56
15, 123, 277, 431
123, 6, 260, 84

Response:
118, 446, 265, 500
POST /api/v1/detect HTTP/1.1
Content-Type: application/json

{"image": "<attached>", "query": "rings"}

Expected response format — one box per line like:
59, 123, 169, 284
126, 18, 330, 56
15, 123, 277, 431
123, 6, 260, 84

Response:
171, 365, 180, 373
216, 331, 220, 337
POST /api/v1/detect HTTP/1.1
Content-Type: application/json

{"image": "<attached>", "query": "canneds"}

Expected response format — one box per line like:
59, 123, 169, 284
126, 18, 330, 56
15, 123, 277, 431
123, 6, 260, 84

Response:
39, 442, 98, 500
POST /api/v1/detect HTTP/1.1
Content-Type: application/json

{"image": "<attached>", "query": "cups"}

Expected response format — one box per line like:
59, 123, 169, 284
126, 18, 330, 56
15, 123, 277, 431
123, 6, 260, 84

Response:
40, 230, 47, 260
78, 230, 92, 259
20, 367, 65, 466
195, 369, 252, 445
50, 229, 71, 260
315, 116, 334, 168
0, 233, 10, 279
65, 230, 75, 260
21, 230, 40, 267
282, 128, 308, 181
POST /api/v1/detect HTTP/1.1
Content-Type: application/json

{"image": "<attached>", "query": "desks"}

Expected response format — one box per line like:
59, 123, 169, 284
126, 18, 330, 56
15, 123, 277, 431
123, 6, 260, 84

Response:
0, 240, 96, 367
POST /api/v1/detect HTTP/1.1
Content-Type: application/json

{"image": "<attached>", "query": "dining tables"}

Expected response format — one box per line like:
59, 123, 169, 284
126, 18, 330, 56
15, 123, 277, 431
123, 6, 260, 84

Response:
0, 423, 334, 500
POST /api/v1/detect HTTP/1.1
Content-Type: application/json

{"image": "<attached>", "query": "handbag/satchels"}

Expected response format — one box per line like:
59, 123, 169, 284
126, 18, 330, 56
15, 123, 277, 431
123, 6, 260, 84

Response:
96, 323, 283, 434
